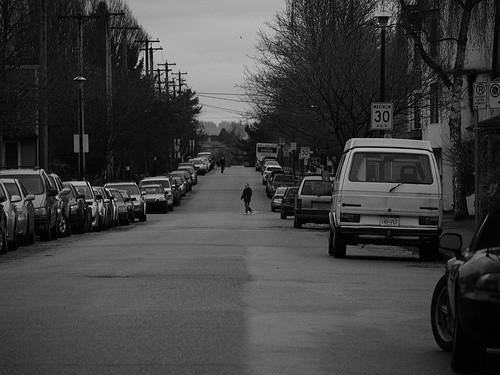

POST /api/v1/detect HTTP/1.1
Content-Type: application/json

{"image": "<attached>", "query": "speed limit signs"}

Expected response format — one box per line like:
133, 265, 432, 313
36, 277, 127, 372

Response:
371, 103, 393, 130
302, 148, 309, 158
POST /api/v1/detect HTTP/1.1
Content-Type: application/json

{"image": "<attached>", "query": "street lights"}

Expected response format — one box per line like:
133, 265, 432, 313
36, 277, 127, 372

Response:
74, 76, 87, 193
190, 120, 195, 157
372, 10, 393, 182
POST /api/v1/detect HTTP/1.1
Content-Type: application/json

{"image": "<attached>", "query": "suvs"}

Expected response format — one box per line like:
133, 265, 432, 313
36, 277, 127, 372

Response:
0, 166, 57, 242
45, 171, 71, 236
103, 181, 147, 222
291, 176, 335, 229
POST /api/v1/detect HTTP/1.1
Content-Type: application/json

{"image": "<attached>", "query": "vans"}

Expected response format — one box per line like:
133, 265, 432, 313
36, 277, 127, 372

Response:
322, 138, 444, 261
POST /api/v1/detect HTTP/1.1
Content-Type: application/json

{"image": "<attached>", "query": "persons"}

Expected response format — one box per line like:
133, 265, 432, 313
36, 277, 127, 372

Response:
240, 183, 255, 216
219, 156, 225, 173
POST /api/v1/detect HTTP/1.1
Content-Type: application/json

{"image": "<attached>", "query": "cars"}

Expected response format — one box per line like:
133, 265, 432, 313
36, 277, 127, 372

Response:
271, 187, 298, 219
139, 152, 214, 213
260, 156, 295, 198
61, 179, 135, 235
430, 192, 500, 375
0, 179, 36, 255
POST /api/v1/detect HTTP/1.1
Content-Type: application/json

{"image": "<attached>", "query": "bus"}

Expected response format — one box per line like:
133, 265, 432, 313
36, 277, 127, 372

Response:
255, 143, 278, 170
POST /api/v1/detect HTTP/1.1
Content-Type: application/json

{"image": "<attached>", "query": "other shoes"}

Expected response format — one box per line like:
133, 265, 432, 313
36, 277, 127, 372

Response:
244, 212, 248, 215
250, 210, 253, 214
